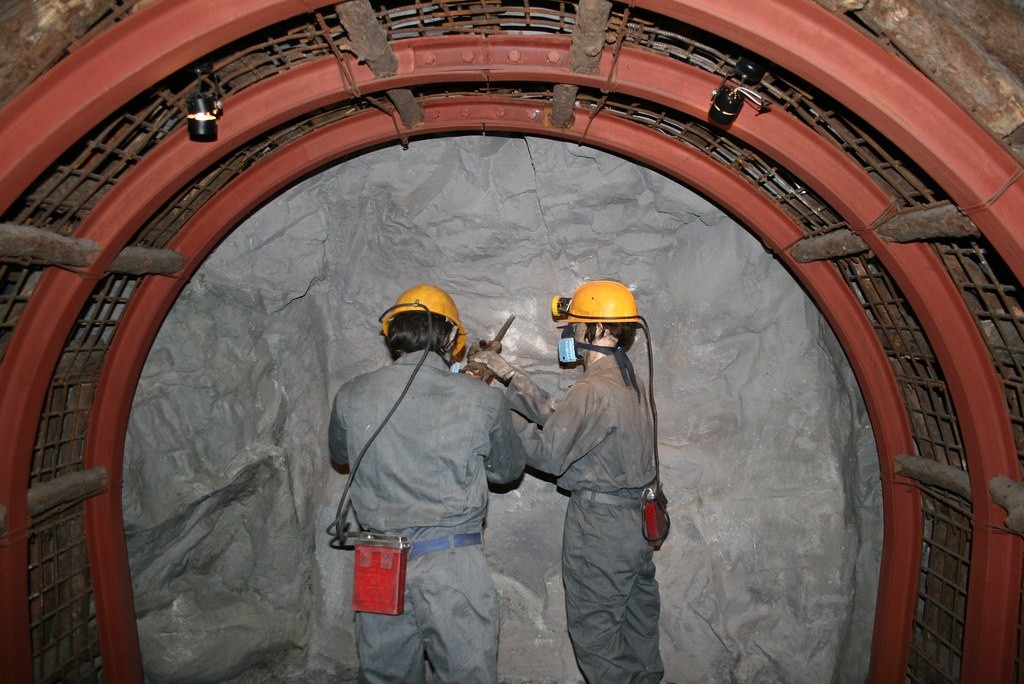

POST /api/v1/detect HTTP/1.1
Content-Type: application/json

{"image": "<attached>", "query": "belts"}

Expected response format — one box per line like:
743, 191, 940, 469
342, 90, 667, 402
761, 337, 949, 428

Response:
572, 488, 644, 508
406, 532, 482, 557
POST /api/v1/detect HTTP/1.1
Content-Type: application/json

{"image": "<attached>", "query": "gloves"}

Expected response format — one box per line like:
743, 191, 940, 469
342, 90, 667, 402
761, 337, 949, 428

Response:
459, 340, 502, 384
468, 350, 515, 382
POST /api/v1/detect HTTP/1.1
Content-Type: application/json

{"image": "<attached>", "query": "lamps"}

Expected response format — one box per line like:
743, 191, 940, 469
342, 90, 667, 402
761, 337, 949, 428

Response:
708, 58, 774, 126
185, 68, 223, 141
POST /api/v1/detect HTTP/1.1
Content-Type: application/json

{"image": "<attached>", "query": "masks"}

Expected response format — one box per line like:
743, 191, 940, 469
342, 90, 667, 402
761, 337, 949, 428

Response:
558, 338, 578, 364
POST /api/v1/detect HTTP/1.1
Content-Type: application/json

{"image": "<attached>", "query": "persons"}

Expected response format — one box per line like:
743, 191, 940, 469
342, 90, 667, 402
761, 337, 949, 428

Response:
326, 283, 527, 684
469, 280, 665, 683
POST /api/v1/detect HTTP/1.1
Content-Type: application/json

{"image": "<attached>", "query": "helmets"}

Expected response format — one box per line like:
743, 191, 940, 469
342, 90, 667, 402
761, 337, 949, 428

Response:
552, 280, 639, 323
382, 284, 466, 356
